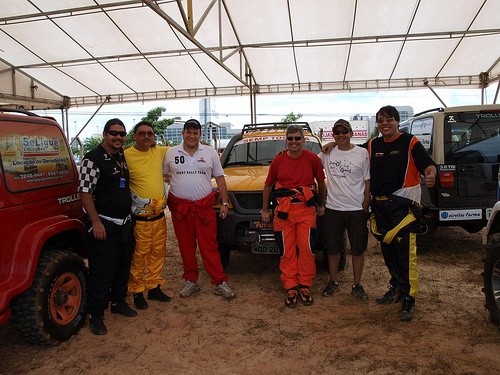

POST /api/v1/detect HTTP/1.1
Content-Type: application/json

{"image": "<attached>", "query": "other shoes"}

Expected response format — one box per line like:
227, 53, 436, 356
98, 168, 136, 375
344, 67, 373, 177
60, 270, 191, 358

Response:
133, 292, 148, 310
148, 284, 171, 301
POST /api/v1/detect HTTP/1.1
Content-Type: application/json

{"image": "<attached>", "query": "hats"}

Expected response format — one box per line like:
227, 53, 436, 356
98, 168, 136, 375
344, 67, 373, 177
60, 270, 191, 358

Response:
184, 119, 201, 130
334, 119, 352, 132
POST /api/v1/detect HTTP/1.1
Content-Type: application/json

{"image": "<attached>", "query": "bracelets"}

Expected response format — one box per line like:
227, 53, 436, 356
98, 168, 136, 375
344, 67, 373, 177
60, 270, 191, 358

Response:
222, 203, 228, 205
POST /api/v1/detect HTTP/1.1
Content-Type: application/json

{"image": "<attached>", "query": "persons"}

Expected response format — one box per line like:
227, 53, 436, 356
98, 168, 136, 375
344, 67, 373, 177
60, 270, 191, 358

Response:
163, 119, 236, 298
262, 125, 325, 307
276, 119, 370, 301
321, 106, 438, 321
122, 122, 210, 309
77, 119, 156, 335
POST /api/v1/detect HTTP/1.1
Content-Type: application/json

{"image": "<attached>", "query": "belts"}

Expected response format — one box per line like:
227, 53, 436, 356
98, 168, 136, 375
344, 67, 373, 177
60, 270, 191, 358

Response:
136, 211, 165, 222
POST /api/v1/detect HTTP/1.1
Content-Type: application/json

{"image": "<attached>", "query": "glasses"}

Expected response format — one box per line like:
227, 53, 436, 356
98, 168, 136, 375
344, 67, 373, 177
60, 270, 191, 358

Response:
287, 136, 302, 141
377, 117, 396, 124
108, 130, 126, 137
136, 131, 156, 138
334, 129, 349, 135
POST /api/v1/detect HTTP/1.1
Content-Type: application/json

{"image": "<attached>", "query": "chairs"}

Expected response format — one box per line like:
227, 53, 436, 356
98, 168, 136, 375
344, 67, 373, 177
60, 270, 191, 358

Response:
452, 132, 468, 153
480, 129, 499, 140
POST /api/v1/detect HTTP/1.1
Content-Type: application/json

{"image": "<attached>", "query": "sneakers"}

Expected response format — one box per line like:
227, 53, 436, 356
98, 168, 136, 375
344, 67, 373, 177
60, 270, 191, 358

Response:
323, 281, 339, 296
285, 288, 297, 307
400, 295, 415, 322
215, 281, 236, 298
110, 302, 138, 317
376, 285, 400, 303
180, 280, 199, 297
352, 283, 368, 300
89, 315, 107, 335
298, 285, 313, 305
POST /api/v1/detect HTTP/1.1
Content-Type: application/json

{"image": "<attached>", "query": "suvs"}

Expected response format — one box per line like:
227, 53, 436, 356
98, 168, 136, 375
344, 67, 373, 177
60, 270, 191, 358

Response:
0, 106, 95, 349
210, 120, 332, 268
397, 103, 500, 240
